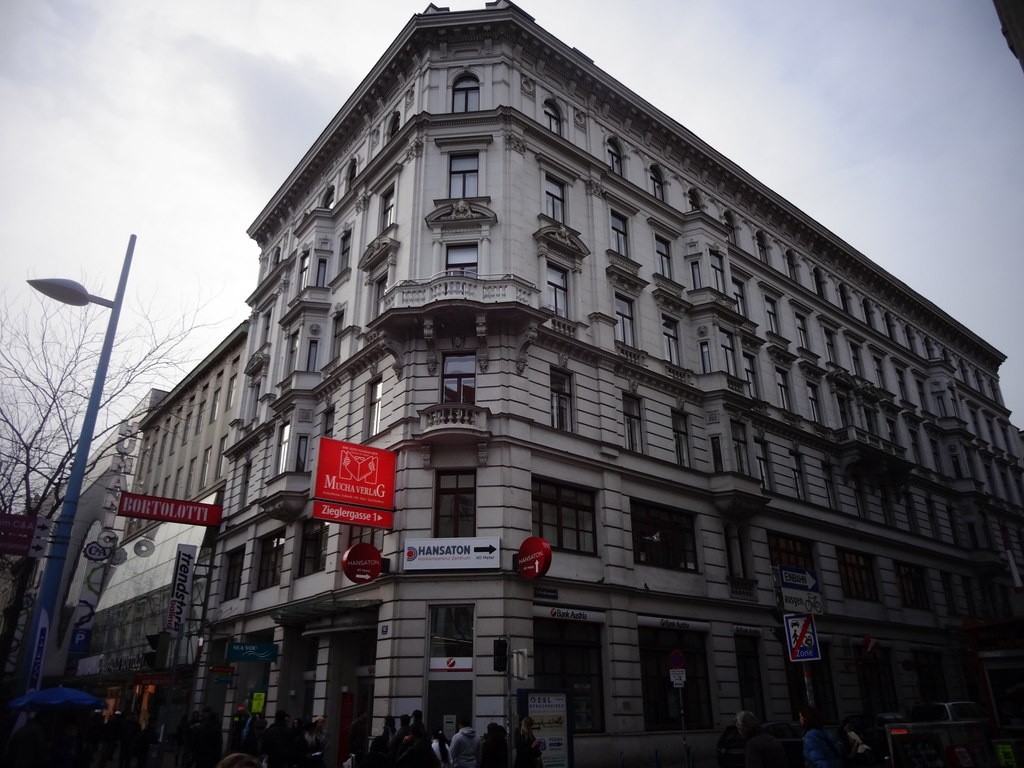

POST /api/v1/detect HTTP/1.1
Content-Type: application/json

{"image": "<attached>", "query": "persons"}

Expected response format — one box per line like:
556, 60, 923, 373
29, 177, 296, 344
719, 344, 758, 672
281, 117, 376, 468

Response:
0, 707, 542, 768
732, 706, 846, 768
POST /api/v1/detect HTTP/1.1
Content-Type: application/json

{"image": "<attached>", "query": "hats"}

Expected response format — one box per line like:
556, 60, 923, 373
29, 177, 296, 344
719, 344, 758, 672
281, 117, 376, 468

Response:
736, 712, 760, 729
256, 720, 267, 728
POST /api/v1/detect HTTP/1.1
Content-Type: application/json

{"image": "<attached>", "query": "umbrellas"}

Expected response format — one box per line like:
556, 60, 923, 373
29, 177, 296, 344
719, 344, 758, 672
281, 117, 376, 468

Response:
9, 686, 108, 720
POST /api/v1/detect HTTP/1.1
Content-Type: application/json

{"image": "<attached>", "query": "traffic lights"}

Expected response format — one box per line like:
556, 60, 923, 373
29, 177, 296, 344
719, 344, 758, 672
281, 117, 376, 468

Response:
492, 639, 508, 673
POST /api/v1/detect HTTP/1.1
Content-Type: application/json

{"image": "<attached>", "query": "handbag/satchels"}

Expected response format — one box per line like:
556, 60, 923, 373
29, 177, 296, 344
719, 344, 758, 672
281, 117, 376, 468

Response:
536, 757, 544, 768
403, 735, 414, 744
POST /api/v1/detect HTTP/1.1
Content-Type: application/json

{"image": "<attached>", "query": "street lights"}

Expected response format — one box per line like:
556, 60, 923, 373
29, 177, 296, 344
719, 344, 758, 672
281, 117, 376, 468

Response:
27, 232, 141, 705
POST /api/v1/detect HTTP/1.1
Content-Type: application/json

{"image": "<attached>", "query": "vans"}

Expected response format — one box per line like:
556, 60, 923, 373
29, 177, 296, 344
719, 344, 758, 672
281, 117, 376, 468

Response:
909, 699, 988, 723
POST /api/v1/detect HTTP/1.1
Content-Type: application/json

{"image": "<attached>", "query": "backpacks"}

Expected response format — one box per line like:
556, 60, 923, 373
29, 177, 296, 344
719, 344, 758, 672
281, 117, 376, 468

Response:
387, 727, 397, 747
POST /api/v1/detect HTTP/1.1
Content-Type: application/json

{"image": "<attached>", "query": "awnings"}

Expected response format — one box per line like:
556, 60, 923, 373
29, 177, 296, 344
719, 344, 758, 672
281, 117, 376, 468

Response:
269, 592, 384, 637
